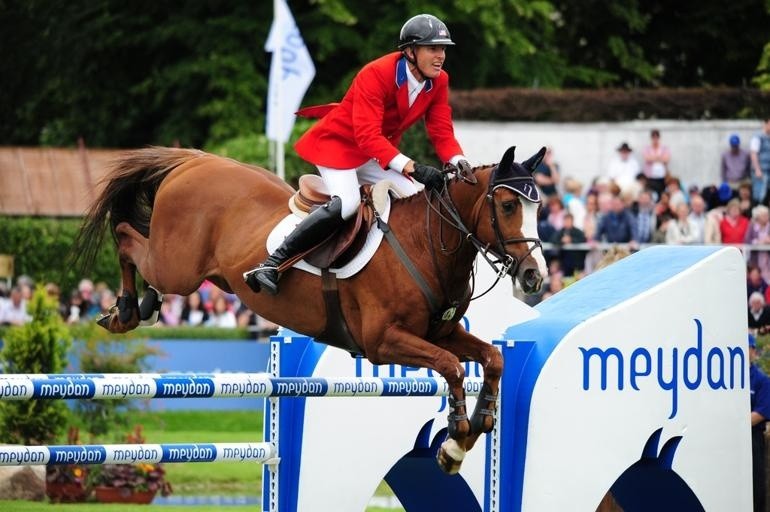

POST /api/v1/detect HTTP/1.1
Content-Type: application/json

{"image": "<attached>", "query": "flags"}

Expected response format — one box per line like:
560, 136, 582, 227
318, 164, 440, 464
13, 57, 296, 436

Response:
263, 0, 318, 142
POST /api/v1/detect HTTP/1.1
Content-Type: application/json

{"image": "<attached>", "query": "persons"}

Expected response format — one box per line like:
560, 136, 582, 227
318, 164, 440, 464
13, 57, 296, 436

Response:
532, 128, 770, 333
749, 336, 770, 512
1, 275, 254, 329
255, 13, 471, 296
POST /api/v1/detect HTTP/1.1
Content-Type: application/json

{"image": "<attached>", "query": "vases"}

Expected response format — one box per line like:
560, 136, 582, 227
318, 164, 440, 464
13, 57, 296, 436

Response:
95, 484, 158, 504
45, 481, 89, 504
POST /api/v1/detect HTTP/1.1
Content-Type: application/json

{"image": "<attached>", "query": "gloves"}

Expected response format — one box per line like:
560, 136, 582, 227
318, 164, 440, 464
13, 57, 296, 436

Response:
409, 162, 444, 190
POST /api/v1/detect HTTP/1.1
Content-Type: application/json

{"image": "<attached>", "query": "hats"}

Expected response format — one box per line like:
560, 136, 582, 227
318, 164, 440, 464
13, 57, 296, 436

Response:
617, 144, 632, 151
719, 184, 731, 200
730, 136, 739, 146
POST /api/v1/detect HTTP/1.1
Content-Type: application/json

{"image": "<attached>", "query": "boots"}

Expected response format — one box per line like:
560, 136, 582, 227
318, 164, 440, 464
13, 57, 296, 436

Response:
254, 196, 346, 294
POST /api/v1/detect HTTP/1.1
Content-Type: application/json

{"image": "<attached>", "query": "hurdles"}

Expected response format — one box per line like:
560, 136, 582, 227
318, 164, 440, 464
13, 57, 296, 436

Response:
0, 342, 501, 511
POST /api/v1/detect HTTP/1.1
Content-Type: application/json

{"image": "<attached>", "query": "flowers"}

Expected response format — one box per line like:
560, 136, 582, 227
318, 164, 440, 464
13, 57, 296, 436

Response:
45, 425, 92, 487
92, 424, 174, 498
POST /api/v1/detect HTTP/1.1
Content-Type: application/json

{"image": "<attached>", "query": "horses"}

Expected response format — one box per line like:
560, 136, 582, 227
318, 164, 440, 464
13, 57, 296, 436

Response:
62, 145, 550, 476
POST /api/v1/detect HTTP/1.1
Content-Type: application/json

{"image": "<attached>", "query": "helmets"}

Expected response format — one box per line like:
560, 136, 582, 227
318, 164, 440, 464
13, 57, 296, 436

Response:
398, 14, 455, 49
